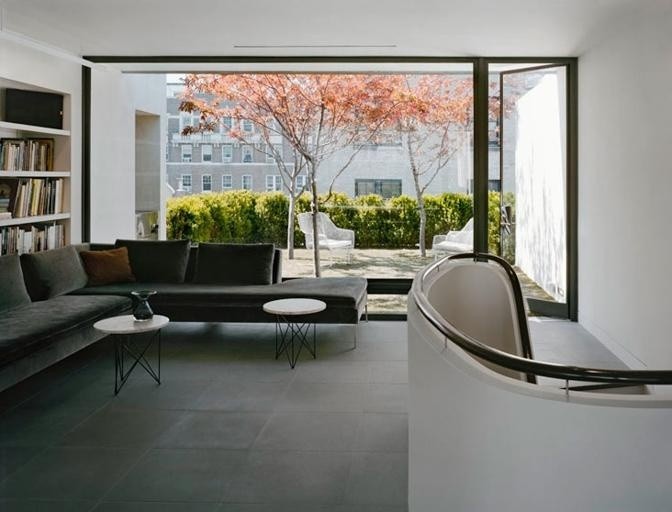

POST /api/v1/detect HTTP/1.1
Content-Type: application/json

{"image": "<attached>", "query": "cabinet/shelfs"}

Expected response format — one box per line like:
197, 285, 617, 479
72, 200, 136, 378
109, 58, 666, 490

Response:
0, 77, 72, 257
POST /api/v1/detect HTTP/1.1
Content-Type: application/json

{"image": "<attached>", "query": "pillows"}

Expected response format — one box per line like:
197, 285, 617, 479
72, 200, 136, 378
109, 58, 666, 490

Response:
79, 247, 135, 287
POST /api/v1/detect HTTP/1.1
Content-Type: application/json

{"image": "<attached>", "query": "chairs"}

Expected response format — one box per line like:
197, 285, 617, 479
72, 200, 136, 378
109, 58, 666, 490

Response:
297, 212, 355, 269
431, 217, 475, 262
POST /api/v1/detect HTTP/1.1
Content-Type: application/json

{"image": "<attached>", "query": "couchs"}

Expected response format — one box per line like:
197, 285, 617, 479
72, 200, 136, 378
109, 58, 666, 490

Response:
0, 243, 368, 394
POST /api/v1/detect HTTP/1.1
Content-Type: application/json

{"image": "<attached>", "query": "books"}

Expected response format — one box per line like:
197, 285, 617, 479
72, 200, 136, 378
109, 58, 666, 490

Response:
0, 138, 54, 171
0, 221, 65, 257
0, 178, 63, 219
